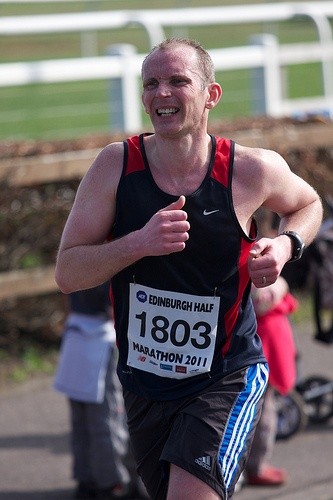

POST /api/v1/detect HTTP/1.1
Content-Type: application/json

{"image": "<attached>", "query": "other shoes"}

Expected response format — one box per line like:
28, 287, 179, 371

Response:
248, 466, 286, 485
78, 479, 130, 498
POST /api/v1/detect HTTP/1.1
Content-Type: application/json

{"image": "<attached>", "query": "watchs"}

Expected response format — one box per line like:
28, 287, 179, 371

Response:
277, 231, 306, 264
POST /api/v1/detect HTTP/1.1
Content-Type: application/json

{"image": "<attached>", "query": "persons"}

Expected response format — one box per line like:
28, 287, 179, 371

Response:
242, 204, 299, 489
58, 278, 132, 500
302, 144, 333, 347
55, 37, 325, 500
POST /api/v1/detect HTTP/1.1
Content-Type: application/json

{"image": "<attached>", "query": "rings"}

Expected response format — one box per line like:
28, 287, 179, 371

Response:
262, 277, 267, 283
